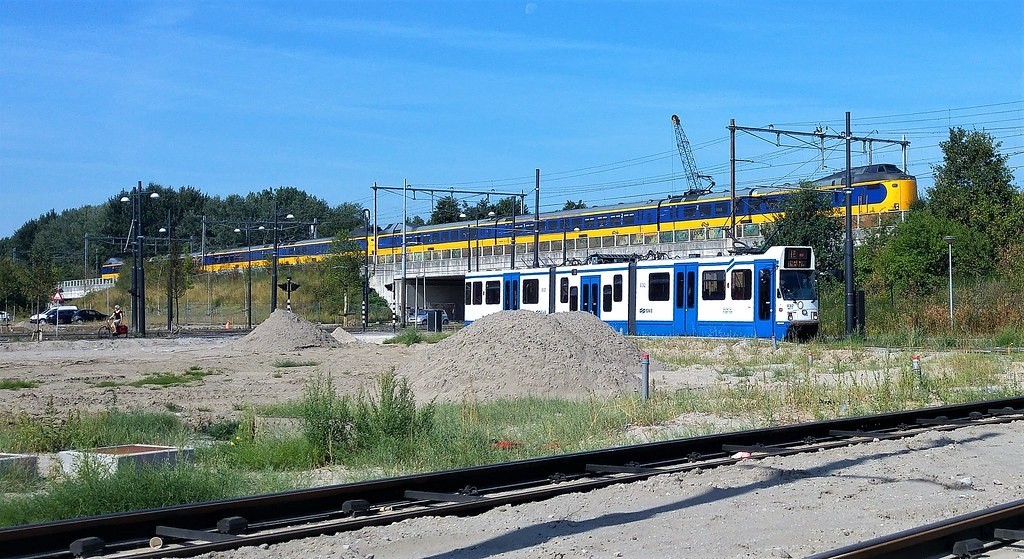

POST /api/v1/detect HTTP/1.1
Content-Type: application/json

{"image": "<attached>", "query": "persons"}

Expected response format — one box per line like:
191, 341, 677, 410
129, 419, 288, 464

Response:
107, 305, 123, 333
705, 285, 742, 300
226, 320, 232, 330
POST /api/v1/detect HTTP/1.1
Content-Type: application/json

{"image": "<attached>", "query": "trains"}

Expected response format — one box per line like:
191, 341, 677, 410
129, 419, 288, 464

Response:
97, 161, 918, 281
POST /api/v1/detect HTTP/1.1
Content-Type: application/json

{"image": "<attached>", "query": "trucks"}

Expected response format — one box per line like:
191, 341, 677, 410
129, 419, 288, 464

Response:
29, 305, 78, 325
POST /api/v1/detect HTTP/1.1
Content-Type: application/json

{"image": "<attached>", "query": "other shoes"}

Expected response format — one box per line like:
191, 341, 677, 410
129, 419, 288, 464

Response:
113, 330, 117, 333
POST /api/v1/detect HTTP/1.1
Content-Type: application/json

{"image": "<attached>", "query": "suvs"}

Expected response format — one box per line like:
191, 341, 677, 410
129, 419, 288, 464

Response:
47, 309, 81, 326
0, 311, 11, 322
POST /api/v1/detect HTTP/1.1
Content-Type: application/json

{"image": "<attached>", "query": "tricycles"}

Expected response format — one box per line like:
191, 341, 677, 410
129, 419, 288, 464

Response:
97, 316, 130, 339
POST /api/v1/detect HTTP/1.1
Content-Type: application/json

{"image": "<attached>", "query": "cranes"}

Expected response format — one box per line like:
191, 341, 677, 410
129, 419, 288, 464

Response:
671, 114, 706, 194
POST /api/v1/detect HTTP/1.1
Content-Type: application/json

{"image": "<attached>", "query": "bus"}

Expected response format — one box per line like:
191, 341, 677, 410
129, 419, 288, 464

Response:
461, 216, 822, 343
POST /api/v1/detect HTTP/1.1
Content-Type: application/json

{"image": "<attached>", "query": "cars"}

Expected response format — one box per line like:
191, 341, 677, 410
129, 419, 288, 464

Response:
408, 308, 449, 325
70, 309, 110, 324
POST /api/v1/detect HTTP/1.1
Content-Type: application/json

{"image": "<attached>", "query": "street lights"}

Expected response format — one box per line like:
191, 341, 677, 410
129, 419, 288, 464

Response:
120, 186, 138, 338
233, 198, 295, 331
943, 235, 957, 331
136, 180, 163, 338
